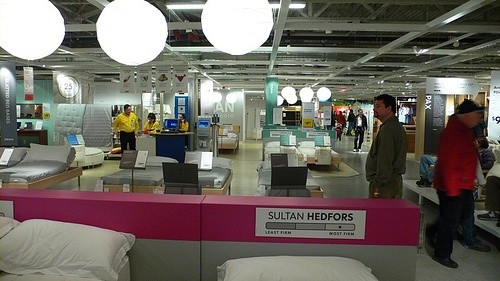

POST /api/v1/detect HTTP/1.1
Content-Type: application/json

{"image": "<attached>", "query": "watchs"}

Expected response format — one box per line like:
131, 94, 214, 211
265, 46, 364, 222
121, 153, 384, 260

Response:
373, 193, 381, 197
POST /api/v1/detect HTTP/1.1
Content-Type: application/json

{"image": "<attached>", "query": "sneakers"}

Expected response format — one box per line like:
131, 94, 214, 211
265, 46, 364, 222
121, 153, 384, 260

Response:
477, 209, 498, 220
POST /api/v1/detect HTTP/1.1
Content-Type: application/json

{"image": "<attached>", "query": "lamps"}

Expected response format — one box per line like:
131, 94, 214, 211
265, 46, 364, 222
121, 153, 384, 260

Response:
0, 0, 66, 61
96, 0, 168, 66
201, 0, 274, 55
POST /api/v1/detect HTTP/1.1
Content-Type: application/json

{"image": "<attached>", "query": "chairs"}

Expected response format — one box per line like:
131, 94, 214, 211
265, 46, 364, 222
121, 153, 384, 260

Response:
473, 145, 497, 201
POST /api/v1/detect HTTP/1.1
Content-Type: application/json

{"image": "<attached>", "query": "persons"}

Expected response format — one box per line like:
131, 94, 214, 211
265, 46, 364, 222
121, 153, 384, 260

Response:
425, 98, 486, 268
114, 104, 139, 157
364, 94, 408, 199
145, 113, 160, 134
460, 158, 490, 253
354, 109, 368, 152
179, 112, 190, 131
477, 176, 500, 228
333, 109, 356, 141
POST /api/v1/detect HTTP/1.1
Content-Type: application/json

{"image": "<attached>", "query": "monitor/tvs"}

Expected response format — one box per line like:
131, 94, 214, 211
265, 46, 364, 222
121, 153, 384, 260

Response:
164, 118, 179, 130
198, 119, 210, 128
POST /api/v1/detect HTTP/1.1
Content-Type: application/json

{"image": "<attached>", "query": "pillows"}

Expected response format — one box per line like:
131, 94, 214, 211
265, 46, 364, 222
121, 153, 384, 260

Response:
146, 156, 178, 166
20, 143, 71, 163
0, 219, 136, 281
216, 256, 379, 280
0, 216, 21, 240
0, 147, 27, 161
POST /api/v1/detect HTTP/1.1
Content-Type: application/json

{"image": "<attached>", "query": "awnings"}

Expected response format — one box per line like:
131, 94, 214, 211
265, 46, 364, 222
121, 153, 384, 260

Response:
416, 137, 497, 189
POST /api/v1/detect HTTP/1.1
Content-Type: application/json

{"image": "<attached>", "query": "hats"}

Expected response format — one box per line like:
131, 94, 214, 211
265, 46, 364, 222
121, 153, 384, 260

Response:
454, 99, 484, 115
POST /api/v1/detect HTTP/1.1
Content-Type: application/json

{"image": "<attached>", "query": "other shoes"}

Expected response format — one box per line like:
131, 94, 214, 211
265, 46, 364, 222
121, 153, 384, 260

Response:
463, 239, 491, 252
431, 254, 458, 268
353, 149, 356, 152
357, 149, 362, 152
416, 179, 432, 188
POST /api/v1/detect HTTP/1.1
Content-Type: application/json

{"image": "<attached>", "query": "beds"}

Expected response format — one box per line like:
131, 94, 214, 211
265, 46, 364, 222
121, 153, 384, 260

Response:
184, 124, 341, 197
0, 148, 82, 190
1, 255, 131, 281
0, 147, 29, 169
100, 166, 165, 194
